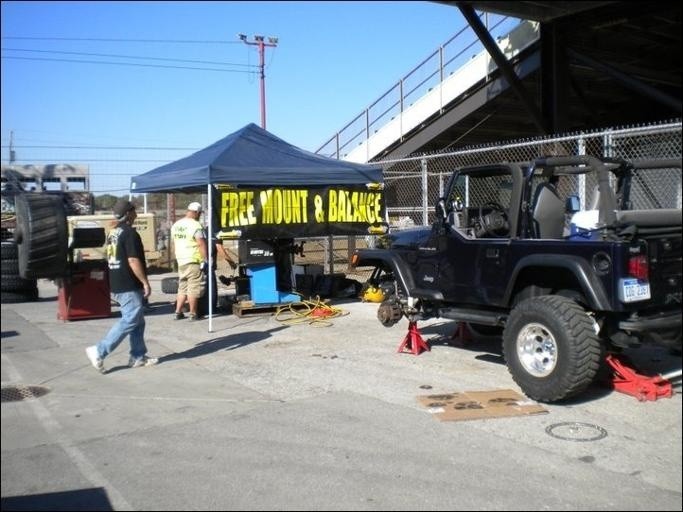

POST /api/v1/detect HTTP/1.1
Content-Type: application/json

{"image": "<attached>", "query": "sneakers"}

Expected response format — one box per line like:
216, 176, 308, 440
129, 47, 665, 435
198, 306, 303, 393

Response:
128, 354, 158, 366
173, 311, 204, 321
86, 346, 106, 374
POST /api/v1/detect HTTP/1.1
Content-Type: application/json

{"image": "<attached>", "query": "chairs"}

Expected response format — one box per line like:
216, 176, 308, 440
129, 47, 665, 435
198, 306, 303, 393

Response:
516, 180, 683, 239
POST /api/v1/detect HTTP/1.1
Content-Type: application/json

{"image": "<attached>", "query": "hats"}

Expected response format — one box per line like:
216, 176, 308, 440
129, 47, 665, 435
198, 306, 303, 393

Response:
188, 202, 205, 212
113, 199, 135, 220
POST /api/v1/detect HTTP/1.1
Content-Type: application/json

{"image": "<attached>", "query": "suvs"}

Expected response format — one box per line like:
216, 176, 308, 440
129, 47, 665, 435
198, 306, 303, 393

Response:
347, 152, 683, 406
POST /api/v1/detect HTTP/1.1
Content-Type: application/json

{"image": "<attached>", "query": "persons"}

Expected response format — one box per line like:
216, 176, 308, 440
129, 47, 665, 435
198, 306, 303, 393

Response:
198, 224, 237, 315
85, 201, 160, 374
170, 201, 208, 321
